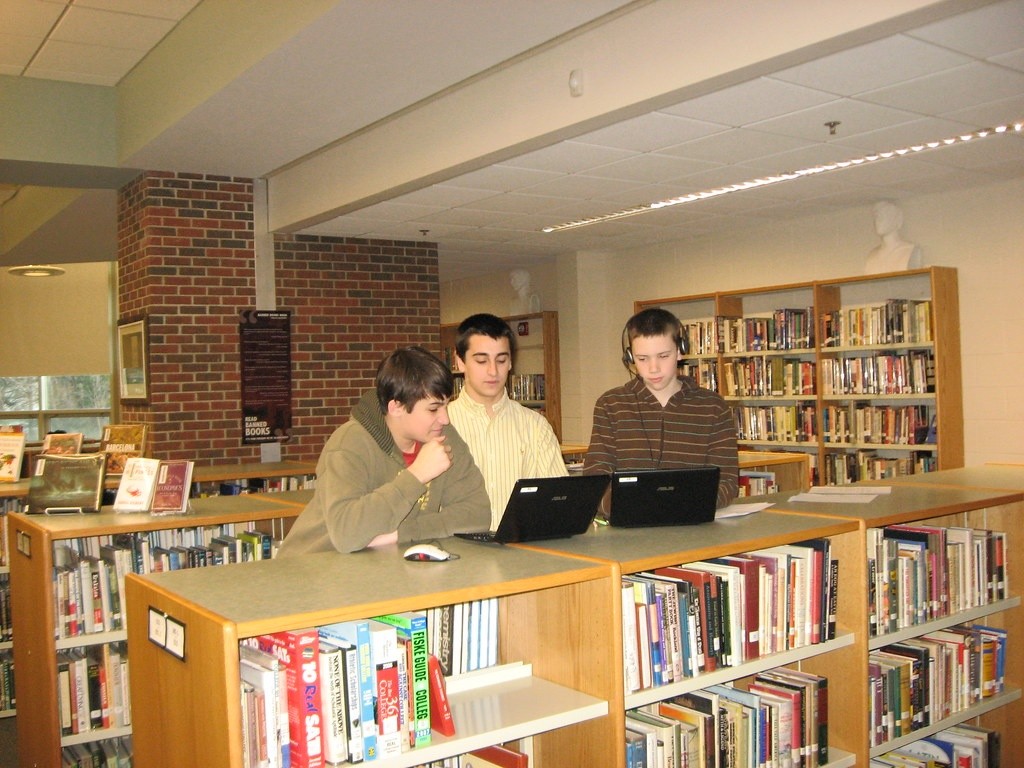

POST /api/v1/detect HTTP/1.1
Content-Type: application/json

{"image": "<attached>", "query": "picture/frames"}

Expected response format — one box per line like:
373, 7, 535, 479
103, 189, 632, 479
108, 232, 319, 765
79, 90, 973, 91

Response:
114, 313, 150, 406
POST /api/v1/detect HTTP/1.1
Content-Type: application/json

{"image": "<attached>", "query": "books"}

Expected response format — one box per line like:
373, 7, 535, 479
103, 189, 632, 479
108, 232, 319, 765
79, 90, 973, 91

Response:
1, 423, 528, 768
679, 297, 939, 498
449, 373, 547, 403
620, 524, 1009, 768
565, 458, 585, 472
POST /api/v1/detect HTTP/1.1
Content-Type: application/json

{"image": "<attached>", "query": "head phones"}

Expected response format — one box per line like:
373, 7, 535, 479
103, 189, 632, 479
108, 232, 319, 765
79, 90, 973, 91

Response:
621, 309, 690, 365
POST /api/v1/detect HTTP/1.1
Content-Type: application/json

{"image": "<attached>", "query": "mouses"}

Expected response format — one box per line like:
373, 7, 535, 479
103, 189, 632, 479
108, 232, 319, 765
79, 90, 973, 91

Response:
403, 545, 451, 562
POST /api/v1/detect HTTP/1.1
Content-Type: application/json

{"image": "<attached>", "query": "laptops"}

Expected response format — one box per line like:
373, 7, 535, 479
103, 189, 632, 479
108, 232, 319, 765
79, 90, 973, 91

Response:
610, 466, 722, 528
454, 474, 611, 543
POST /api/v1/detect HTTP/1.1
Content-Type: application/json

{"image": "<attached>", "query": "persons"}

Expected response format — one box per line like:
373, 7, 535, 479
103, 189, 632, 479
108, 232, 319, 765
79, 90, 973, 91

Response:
584, 308, 739, 517
275, 346, 491, 559
446, 313, 569, 533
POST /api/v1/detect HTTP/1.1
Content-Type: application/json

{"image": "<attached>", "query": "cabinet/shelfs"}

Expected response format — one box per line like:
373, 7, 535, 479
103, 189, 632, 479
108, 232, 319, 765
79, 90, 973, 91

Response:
0, 445, 1024, 768
440, 311, 562, 445
634, 266, 964, 487
